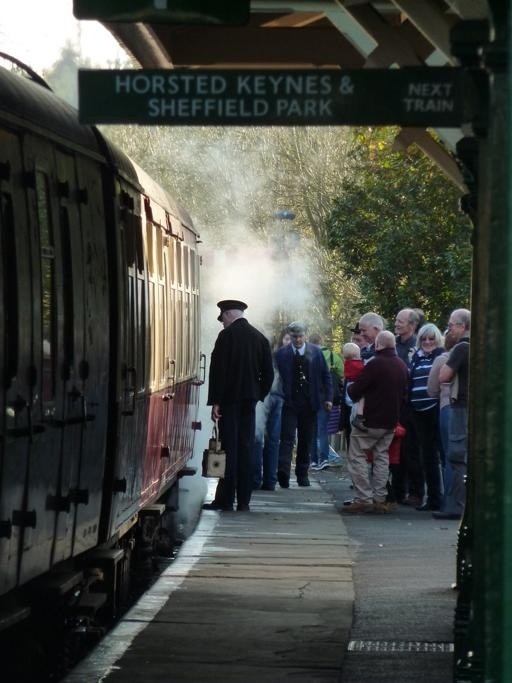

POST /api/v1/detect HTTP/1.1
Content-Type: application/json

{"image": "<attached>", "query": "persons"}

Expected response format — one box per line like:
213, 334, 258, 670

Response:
340, 307, 470, 519
201, 298, 275, 512
250, 321, 344, 492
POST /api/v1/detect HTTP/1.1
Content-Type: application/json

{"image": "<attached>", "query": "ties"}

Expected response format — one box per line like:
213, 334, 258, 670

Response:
296, 350, 309, 397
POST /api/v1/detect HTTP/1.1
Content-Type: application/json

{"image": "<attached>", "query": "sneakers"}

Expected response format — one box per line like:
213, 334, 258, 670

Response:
310, 458, 344, 472
201, 501, 234, 510
342, 493, 400, 515
253, 478, 290, 492
236, 502, 250, 512
415, 502, 442, 512
398, 494, 425, 508
432, 511, 462, 521
297, 478, 311, 487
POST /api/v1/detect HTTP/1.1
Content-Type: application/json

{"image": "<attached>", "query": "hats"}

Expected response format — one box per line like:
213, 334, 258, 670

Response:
216, 300, 248, 322
349, 322, 361, 334
288, 321, 307, 337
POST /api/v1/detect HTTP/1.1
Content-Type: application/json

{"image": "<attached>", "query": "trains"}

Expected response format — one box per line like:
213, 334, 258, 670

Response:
0, 51, 206, 632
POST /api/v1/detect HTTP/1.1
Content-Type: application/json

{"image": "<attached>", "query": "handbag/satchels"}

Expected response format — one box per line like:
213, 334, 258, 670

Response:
329, 351, 343, 406
202, 418, 227, 480
